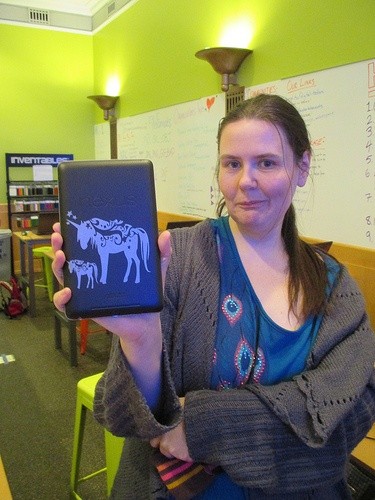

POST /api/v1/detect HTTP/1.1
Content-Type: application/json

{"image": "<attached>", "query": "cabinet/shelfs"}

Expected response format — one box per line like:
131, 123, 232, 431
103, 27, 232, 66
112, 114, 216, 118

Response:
5, 153, 73, 276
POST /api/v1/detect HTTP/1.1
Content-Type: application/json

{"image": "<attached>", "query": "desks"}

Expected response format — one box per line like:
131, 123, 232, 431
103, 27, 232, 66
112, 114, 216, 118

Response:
13, 231, 51, 315
46, 252, 79, 366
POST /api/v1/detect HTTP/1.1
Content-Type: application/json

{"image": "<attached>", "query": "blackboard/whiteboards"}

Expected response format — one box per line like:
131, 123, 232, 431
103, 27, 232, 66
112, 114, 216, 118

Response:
94, 92, 229, 220
245, 58, 375, 248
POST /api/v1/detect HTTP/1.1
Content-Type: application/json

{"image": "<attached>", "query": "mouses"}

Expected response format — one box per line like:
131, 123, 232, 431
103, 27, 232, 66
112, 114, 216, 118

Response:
21, 231, 27, 236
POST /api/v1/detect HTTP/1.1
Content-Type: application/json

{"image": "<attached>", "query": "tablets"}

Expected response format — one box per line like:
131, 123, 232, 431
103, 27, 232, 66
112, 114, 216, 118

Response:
56, 160, 163, 320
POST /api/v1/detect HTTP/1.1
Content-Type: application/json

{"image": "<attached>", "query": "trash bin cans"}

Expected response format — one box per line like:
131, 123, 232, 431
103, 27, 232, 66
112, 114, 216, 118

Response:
0, 228, 13, 280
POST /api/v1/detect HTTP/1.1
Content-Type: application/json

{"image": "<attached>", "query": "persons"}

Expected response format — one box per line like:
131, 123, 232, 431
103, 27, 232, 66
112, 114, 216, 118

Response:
51, 94, 375, 500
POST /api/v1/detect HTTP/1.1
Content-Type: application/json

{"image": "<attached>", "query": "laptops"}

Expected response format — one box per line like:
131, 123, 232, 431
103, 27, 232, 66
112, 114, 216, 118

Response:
31, 211, 59, 236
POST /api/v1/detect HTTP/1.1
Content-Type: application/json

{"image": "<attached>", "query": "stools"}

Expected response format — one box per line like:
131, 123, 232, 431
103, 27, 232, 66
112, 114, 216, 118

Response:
70, 370, 125, 500
27, 249, 111, 355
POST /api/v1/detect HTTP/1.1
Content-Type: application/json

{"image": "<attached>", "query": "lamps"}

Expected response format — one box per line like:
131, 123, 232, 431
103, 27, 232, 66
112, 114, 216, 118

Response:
195, 47, 252, 117
86, 95, 119, 158
